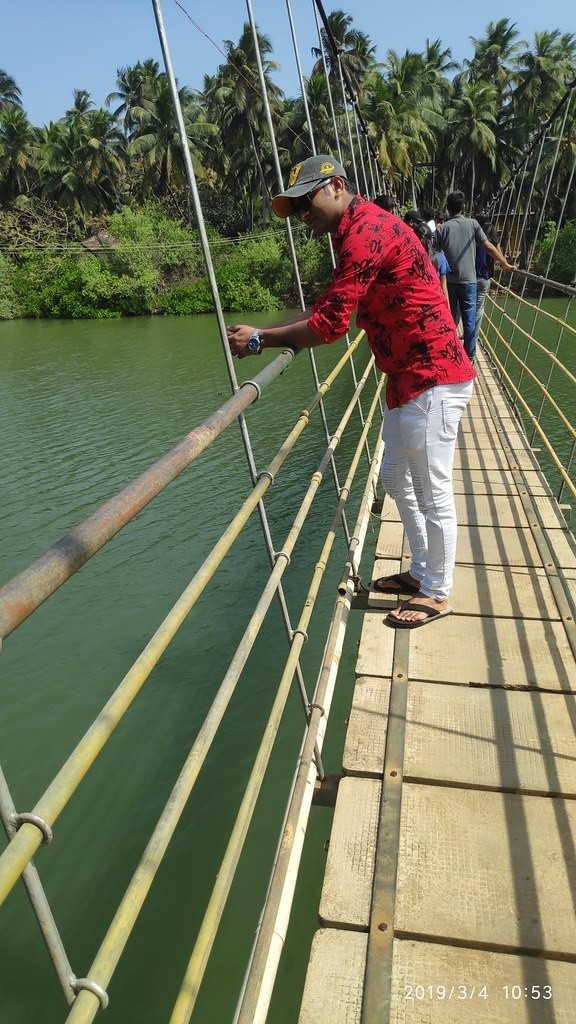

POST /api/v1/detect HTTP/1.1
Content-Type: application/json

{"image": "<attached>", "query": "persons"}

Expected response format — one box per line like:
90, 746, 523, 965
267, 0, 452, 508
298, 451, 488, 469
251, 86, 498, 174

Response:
435, 191, 518, 369
371, 195, 451, 313
456, 215, 507, 339
217, 155, 478, 629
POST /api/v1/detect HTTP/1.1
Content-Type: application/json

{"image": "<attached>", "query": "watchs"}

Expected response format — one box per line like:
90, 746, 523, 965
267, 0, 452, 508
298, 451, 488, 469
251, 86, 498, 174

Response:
246, 326, 262, 356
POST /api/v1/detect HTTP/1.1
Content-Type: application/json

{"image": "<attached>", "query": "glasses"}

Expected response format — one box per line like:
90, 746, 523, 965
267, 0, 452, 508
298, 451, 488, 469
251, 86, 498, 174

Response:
413, 219, 421, 224
288, 178, 334, 214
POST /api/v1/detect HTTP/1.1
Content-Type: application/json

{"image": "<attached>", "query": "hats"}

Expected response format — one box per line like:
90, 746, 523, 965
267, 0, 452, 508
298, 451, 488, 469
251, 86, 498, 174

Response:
403, 211, 423, 222
271, 155, 347, 218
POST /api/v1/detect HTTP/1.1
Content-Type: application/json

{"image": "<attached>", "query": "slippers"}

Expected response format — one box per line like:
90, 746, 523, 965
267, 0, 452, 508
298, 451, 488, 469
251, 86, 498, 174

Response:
468, 359, 478, 368
386, 596, 450, 628
373, 571, 432, 595
